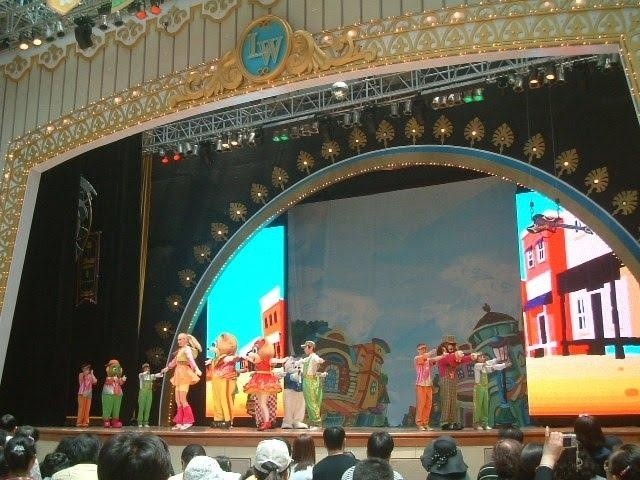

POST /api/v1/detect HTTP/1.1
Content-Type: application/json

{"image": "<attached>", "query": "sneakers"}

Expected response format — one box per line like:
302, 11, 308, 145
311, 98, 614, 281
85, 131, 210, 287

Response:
419, 426, 434, 431
171, 424, 193, 430
474, 426, 492, 430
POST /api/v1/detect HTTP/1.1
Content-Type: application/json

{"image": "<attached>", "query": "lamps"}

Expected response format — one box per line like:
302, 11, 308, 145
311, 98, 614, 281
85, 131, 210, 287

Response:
151, 50, 621, 164
1, 0, 165, 52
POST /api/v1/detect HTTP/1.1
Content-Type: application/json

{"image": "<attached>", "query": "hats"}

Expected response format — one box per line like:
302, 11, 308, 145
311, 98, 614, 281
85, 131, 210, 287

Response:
301, 341, 315, 347
167, 455, 241, 480
255, 439, 293, 474
421, 436, 467, 474
